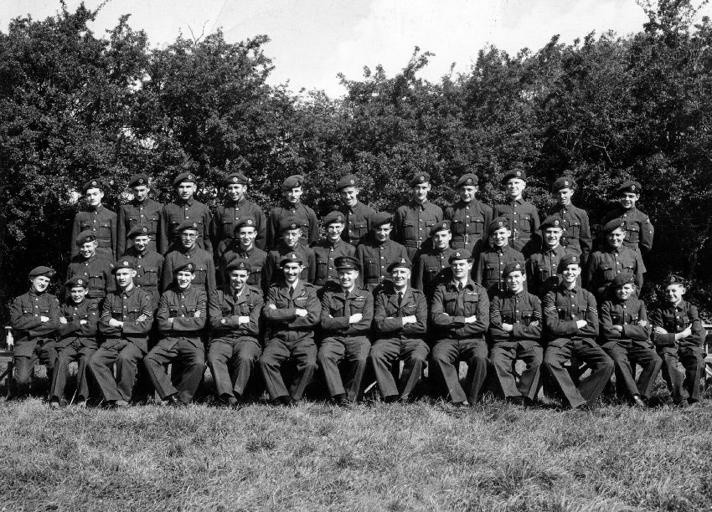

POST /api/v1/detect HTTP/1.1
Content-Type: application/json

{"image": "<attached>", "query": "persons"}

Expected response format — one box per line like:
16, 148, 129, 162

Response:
529, 215, 580, 296
544, 253, 615, 411
491, 262, 543, 409
226, 215, 267, 290
52, 279, 96, 409
208, 261, 261, 407
145, 262, 207, 408
268, 174, 318, 249
417, 220, 460, 294
651, 275, 706, 409
163, 219, 217, 290
66, 229, 112, 298
88, 257, 153, 410
371, 257, 429, 405
476, 216, 527, 298
392, 172, 445, 257
72, 179, 119, 260
162, 172, 212, 252
260, 252, 315, 407
116, 223, 163, 303
594, 181, 655, 273
543, 177, 591, 262
601, 273, 663, 409
589, 220, 645, 304
9, 265, 56, 398
267, 218, 316, 286
118, 173, 161, 255
317, 256, 374, 407
495, 168, 541, 253
327, 174, 377, 248
431, 248, 491, 411
444, 174, 494, 255
212, 173, 268, 253
358, 211, 409, 288
315, 211, 357, 288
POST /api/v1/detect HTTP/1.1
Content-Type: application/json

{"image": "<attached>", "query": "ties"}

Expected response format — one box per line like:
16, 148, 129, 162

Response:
287, 287, 295, 298
343, 290, 350, 301
230, 290, 240, 302
396, 292, 403, 307
455, 282, 464, 291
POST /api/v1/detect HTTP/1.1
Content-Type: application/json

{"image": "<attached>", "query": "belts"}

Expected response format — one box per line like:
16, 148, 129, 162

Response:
404, 239, 425, 248
449, 233, 483, 245
364, 277, 385, 286
558, 237, 580, 246
509, 229, 533, 239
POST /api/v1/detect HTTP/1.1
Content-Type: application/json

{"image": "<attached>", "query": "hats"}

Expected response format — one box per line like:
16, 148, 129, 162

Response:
604, 218, 624, 232
225, 258, 251, 273
279, 250, 305, 269
486, 216, 513, 235
64, 276, 89, 290
550, 176, 579, 193
172, 172, 197, 189
616, 181, 642, 194
279, 216, 304, 234
558, 254, 581, 272
322, 210, 347, 227
81, 181, 105, 193
126, 223, 150, 239
386, 256, 413, 273
500, 169, 527, 185
429, 220, 451, 236
128, 174, 149, 189
455, 173, 480, 187
110, 257, 138, 276
73, 230, 99, 247
334, 255, 363, 271
537, 215, 567, 230
223, 173, 249, 186
502, 261, 527, 277
29, 265, 56, 278
448, 249, 473, 263
612, 273, 636, 285
371, 210, 396, 230
175, 219, 200, 234
409, 172, 431, 187
336, 173, 358, 191
233, 216, 258, 232
282, 174, 305, 191
172, 260, 197, 276
